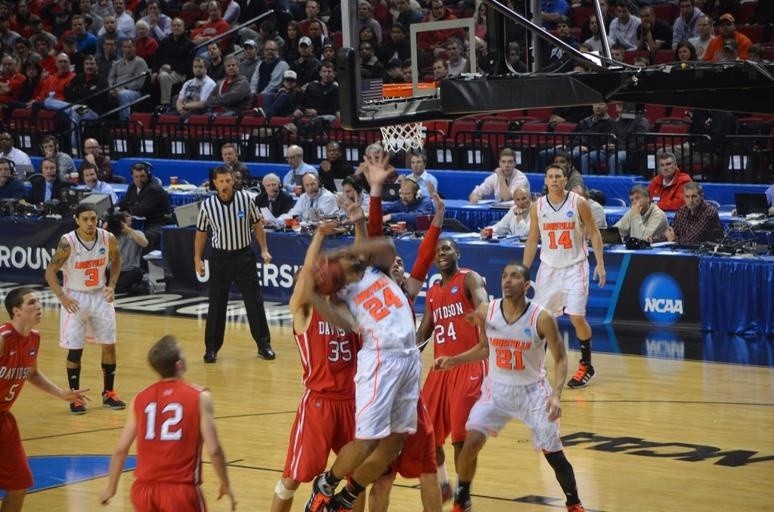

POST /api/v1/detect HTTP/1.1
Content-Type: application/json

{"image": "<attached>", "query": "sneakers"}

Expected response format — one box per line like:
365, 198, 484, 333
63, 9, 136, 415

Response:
452, 482, 472, 512
567, 502, 586, 512
102, 389, 127, 409
441, 482, 452, 502
305, 472, 357, 512
70, 401, 87, 415
567, 359, 596, 388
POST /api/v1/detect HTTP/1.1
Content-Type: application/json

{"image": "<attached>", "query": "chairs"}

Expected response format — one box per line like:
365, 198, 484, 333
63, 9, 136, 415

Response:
0, 0, 773, 183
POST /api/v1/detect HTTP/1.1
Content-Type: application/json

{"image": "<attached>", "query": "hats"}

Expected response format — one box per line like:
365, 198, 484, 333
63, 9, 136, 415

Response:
300, 36, 311, 46
243, 39, 257, 48
283, 70, 297, 81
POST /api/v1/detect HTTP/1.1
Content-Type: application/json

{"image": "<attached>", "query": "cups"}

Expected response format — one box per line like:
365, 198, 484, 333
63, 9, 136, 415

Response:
485, 228, 493, 239
295, 185, 303, 197
397, 220, 408, 232
72, 172, 81, 185
390, 225, 399, 232
170, 176, 179, 184
653, 195, 660, 204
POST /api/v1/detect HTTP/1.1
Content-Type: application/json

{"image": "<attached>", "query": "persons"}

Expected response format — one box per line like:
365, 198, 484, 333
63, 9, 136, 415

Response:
433, 264, 587, 512
193, 167, 277, 363
365, 151, 446, 512
0, 287, 90, 511
103, 210, 148, 293
99, 335, 237, 512
1, 2, 774, 250
268, 191, 368, 512
414, 238, 489, 504
521, 163, 605, 388
46, 203, 126, 414
304, 236, 420, 512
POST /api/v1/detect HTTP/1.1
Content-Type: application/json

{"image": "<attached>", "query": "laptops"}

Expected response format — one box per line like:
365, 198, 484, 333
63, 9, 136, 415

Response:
588, 227, 624, 245
734, 191, 769, 218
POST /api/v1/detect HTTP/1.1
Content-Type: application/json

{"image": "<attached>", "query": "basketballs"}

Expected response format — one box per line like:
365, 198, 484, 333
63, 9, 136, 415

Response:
313, 260, 345, 295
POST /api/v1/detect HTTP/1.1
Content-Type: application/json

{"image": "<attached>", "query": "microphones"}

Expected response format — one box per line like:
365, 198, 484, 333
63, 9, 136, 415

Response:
274, 190, 278, 193
314, 189, 320, 193
91, 179, 98, 182
52, 174, 57, 177
141, 181, 149, 183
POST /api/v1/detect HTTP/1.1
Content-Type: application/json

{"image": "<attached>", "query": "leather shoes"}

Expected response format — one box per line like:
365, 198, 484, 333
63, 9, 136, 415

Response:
259, 347, 274, 358
204, 350, 216, 362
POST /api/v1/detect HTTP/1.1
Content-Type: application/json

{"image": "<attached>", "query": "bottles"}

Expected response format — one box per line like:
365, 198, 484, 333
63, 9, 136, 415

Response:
291, 214, 302, 232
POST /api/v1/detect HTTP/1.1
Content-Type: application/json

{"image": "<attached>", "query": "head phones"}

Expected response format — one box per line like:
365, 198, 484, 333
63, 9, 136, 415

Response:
398, 177, 424, 200
137, 162, 153, 179
39, 158, 61, 173
0, 156, 16, 174
260, 176, 282, 190
80, 163, 101, 181
39, 136, 60, 155
349, 176, 362, 192
301, 171, 322, 193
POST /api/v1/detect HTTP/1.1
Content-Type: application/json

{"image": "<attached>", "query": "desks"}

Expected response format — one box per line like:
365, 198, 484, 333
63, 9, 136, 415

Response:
0, 175, 773, 336
